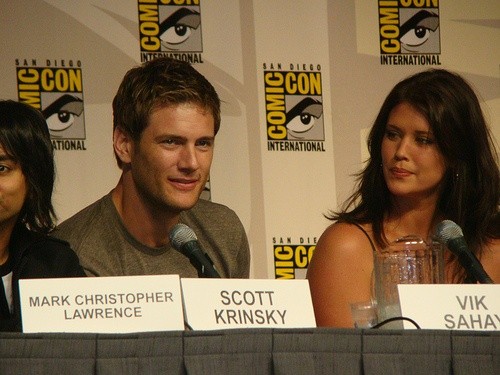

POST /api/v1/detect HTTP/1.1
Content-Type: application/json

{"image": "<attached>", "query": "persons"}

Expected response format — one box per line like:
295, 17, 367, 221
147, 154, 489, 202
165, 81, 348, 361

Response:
48, 57, 252, 279
302, 68, 500, 331
0, 98, 86, 335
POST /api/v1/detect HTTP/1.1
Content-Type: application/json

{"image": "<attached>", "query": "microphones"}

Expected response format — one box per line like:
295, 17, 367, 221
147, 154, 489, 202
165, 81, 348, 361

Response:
436, 220, 494, 284
169, 221, 221, 279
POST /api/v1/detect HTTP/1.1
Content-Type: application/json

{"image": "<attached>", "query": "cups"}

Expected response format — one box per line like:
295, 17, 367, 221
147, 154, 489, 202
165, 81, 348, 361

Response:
352, 301, 377, 330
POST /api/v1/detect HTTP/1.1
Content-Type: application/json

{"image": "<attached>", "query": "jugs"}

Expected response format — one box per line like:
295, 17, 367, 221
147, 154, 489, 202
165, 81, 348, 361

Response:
372, 235, 445, 332
